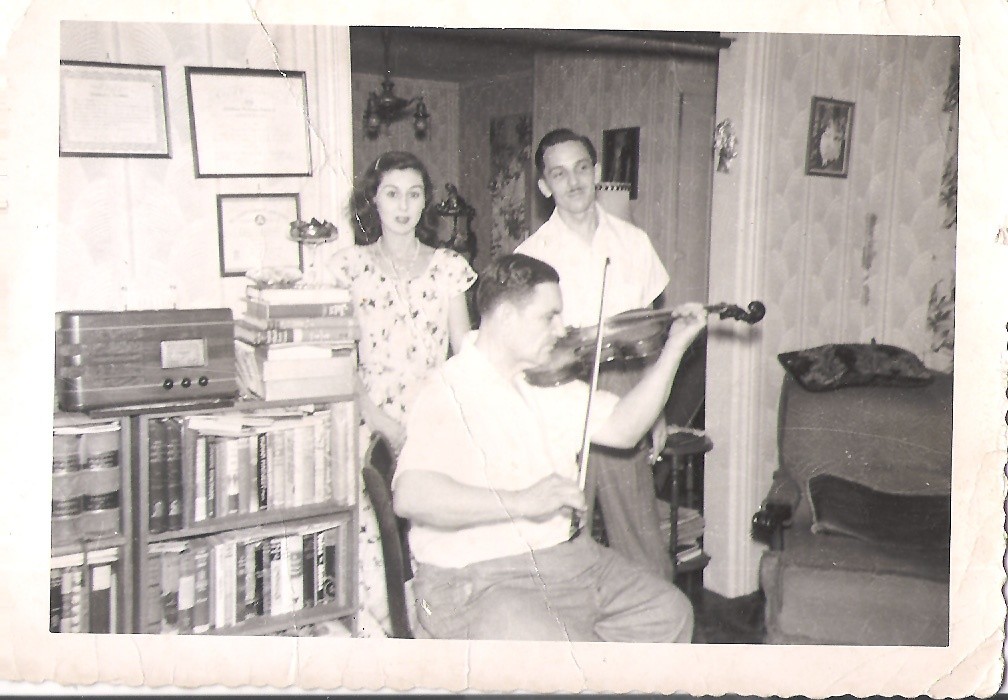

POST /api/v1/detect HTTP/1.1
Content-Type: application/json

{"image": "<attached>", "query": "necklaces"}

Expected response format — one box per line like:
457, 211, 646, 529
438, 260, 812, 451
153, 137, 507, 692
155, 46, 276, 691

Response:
377, 233, 421, 270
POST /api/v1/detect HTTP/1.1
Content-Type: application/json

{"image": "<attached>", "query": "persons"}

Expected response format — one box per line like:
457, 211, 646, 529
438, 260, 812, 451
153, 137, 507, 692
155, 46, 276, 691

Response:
513, 127, 671, 589
388, 252, 709, 640
246, 151, 478, 639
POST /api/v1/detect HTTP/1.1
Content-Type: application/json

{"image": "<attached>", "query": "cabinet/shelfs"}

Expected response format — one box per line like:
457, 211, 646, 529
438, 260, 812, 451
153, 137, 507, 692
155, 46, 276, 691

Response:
49, 390, 362, 637
659, 433, 713, 589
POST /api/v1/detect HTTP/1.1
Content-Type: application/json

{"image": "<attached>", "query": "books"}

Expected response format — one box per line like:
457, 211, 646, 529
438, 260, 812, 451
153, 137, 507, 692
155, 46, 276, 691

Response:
50, 281, 358, 638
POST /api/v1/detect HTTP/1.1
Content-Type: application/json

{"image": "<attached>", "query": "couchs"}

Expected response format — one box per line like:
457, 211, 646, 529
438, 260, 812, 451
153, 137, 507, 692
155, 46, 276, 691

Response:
751, 373, 952, 645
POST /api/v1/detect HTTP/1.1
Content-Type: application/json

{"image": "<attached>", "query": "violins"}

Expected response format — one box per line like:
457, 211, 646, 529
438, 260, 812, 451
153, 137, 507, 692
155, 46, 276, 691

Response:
522, 300, 766, 388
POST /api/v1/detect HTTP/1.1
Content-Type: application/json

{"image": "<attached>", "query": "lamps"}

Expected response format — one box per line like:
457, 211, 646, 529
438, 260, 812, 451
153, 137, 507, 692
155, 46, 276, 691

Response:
596, 182, 634, 226
362, 30, 431, 135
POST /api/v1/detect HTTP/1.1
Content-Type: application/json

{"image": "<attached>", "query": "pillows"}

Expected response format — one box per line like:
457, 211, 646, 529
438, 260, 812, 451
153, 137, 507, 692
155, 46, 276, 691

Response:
807, 474, 950, 552
777, 342, 932, 393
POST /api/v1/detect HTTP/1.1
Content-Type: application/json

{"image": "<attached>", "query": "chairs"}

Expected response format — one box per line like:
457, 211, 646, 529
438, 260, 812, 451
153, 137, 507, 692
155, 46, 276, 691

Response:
362, 432, 415, 639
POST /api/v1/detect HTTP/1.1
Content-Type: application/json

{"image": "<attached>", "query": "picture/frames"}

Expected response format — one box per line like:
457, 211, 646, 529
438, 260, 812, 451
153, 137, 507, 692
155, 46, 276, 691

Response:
804, 96, 856, 178
184, 66, 313, 179
217, 192, 303, 278
